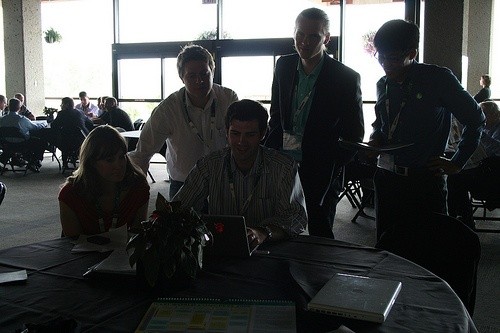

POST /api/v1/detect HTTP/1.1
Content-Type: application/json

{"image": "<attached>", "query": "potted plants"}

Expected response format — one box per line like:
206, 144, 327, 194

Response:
44, 107, 59, 122
44, 27, 62, 44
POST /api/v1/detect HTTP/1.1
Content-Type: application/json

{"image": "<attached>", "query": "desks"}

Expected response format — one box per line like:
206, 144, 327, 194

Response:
0, 234, 479, 333
120, 130, 170, 182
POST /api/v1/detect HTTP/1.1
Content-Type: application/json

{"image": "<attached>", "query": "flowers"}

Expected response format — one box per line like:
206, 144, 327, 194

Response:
125, 190, 225, 280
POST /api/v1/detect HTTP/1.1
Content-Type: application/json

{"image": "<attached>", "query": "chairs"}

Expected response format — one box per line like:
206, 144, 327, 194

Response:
374, 210, 481, 318
0, 126, 83, 176
125, 136, 156, 183
339, 149, 376, 224
462, 157, 500, 236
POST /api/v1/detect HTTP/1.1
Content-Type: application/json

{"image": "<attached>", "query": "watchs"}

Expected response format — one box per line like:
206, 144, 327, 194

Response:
258, 224, 273, 242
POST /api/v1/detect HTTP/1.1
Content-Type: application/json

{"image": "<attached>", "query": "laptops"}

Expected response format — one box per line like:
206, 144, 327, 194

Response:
200, 214, 260, 259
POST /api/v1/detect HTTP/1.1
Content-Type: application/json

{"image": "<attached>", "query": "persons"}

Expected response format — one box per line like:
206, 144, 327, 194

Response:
364, 20, 486, 264
447, 101, 500, 233
171, 98, 308, 243
0, 91, 134, 173
261, 8, 365, 241
58, 124, 151, 239
127, 44, 239, 202
473, 74, 492, 104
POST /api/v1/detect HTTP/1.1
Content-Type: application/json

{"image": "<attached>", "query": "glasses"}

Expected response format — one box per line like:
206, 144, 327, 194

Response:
374, 50, 411, 65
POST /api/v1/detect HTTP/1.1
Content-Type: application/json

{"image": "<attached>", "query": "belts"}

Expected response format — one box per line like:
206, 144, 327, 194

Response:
389, 164, 417, 177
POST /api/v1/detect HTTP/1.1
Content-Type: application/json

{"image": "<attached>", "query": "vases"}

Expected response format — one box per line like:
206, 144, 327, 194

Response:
133, 247, 166, 287
